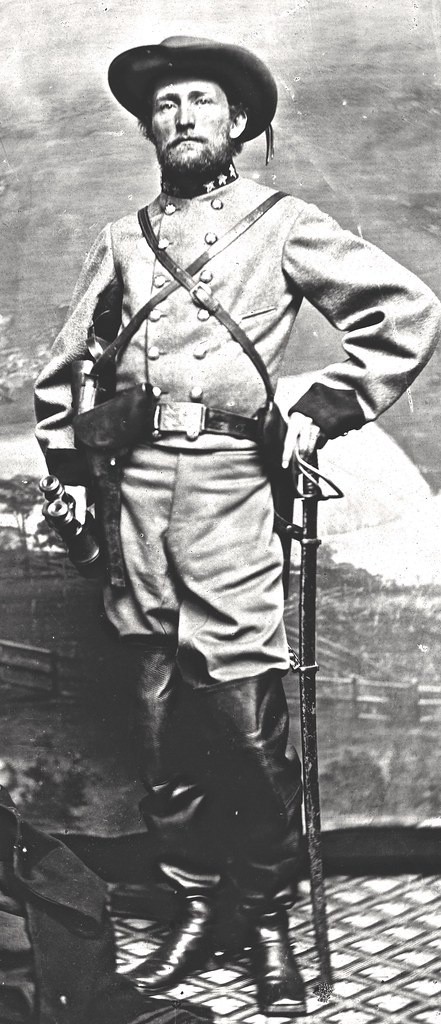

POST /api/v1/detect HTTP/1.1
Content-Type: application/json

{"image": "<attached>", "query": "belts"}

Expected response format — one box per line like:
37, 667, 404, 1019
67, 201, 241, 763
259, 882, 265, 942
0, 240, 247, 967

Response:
144, 399, 255, 440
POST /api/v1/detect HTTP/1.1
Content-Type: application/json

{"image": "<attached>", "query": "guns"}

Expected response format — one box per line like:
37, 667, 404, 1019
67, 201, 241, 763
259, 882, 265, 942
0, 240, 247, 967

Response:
70, 357, 98, 415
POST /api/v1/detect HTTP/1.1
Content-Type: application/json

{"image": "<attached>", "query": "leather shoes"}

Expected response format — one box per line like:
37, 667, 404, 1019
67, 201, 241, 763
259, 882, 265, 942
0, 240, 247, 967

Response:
250, 912, 307, 1015
124, 901, 225, 995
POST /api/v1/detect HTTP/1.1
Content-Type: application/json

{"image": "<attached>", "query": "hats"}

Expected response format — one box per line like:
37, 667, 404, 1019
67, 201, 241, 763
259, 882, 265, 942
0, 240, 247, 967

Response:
108, 35, 278, 165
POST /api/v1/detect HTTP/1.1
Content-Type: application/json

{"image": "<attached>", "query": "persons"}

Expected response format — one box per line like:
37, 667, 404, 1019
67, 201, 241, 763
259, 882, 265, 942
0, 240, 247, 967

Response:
31, 37, 441, 1017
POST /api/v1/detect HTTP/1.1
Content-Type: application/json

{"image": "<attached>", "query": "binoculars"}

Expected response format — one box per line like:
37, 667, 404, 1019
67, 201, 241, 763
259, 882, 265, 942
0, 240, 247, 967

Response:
39, 475, 105, 578
